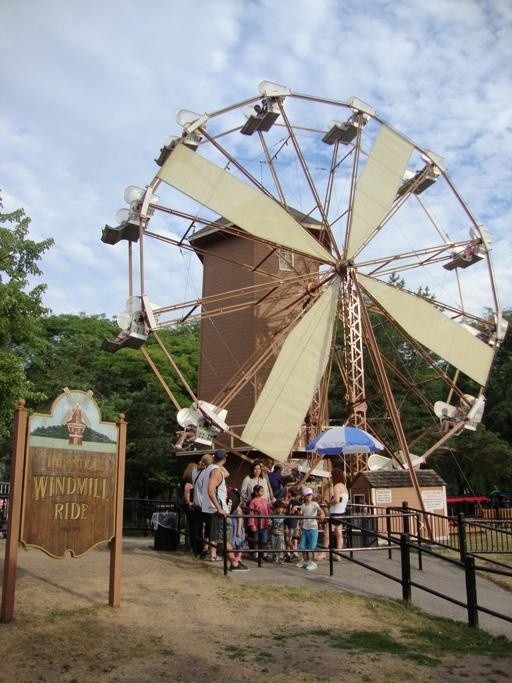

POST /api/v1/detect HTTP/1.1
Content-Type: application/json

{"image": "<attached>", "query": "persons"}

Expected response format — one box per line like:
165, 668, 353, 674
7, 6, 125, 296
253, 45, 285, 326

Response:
171, 430, 196, 449
178, 446, 349, 571
116, 330, 129, 342
464, 242, 475, 258
254, 104, 263, 115
439, 404, 464, 434
476, 322, 492, 343
262, 99, 267, 105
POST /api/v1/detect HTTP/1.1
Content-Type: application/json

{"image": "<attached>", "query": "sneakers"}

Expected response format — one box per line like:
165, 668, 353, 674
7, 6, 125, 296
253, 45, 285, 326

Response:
230, 560, 249, 570
295, 560, 308, 567
306, 561, 317, 570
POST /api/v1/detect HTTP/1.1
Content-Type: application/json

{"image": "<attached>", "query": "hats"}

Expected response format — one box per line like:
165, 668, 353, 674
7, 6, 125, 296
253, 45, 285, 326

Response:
214, 449, 228, 460
302, 488, 313, 497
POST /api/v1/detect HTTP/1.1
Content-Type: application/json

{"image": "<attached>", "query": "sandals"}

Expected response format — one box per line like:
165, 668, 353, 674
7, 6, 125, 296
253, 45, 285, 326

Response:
210, 556, 224, 561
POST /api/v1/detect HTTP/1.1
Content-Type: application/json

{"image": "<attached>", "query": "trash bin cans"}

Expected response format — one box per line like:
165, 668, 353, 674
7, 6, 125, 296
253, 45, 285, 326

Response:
153, 512, 178, 551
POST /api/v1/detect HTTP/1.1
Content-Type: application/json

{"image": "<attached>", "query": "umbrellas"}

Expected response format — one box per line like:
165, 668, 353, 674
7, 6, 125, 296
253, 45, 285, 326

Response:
305, 425, 383, 484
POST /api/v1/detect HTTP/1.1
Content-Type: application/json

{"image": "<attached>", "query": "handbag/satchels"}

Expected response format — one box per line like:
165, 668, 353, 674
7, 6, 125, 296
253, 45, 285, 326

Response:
332, 496, 342, 501
